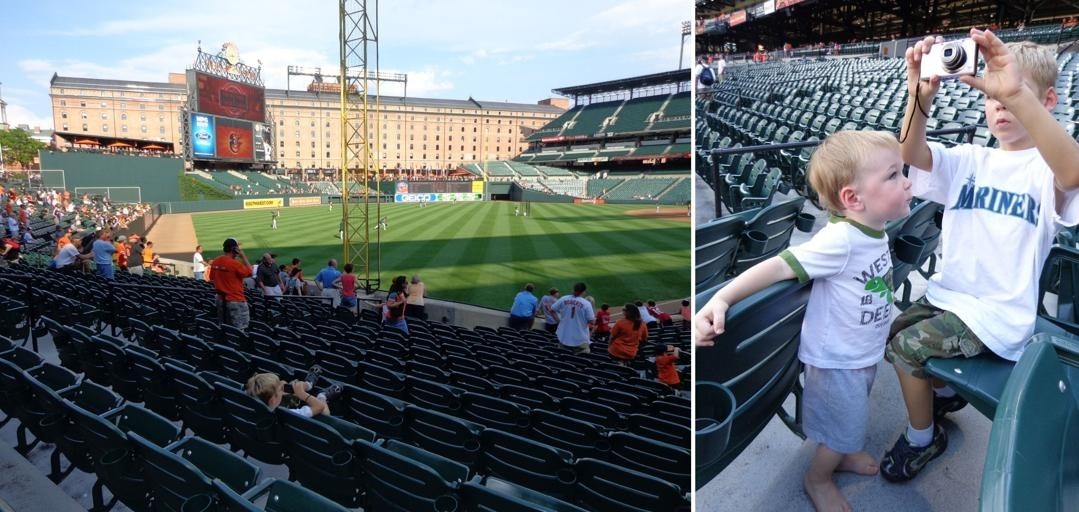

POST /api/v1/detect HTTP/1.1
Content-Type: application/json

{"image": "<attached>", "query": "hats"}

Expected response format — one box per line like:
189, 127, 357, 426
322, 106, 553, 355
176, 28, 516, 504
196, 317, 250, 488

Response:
575, 283, 585, 291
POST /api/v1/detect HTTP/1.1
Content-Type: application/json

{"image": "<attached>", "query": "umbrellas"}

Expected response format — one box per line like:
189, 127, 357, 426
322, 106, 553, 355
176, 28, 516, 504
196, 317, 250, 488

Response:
109, 142, 132, 147
76, 139, 102, 145
143, 144, 164, 149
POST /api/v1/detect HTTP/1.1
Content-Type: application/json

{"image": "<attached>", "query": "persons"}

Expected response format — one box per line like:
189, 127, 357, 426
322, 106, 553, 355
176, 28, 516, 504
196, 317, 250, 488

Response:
695, 130, 913, 512
261, 138, 272, 161
880, 27, 1079, 482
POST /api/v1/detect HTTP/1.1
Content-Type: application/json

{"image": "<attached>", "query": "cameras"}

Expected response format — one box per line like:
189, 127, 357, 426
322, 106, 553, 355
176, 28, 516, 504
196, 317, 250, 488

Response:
283, 384, 294, 393
920, 38, 979, 82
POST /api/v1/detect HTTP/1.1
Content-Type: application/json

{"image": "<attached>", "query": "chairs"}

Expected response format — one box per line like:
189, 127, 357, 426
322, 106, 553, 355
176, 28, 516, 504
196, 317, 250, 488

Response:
202, 162, 387, 202
1, 147, 146, 511
145, 272, 692, 512
462, 92, 691, 202
696, 17, 1078, 512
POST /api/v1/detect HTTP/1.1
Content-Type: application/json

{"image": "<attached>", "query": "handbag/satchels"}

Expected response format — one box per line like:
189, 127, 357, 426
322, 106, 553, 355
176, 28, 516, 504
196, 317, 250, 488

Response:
390, 303, 404, 316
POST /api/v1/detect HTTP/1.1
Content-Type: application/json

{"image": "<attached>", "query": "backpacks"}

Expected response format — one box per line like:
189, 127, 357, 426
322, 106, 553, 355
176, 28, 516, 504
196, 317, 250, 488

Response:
700, 63, 713, 85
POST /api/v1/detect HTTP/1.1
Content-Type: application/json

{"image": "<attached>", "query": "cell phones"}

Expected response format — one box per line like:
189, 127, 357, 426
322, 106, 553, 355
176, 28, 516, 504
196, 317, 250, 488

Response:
232, 248, 240, 254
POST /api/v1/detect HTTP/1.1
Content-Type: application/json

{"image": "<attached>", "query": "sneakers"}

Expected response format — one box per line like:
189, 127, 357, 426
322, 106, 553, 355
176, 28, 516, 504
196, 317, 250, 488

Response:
305, 364, 321, 389
317, 383, 343, 399
880, 391, 968, 483
